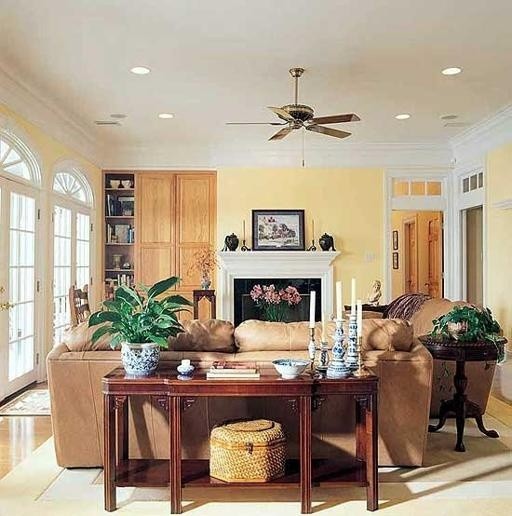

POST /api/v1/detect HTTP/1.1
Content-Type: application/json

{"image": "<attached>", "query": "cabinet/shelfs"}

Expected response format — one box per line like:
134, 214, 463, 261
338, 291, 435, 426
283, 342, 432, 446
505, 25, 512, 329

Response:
99, 172, 216, 321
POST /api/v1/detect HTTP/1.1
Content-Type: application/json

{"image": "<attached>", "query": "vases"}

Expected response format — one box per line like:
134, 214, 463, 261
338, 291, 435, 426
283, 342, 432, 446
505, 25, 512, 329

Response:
201, 275, 212, 290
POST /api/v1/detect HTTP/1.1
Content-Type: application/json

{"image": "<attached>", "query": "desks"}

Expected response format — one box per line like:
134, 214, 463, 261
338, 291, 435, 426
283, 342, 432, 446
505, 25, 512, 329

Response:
419, 333, 507, 452
344, 303, 390, 313
102, 361, 379, 513
193, 290, 216, 320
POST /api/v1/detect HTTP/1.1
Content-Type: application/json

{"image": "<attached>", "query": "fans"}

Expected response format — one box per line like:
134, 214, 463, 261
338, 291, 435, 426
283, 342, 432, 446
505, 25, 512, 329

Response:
225, 68, 360, 141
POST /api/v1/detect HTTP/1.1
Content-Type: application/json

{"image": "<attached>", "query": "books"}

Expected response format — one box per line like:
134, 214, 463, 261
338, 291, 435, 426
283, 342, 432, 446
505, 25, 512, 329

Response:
106, 223, 134, 243
210, 360, 256, 374
117, 274, 134, 289
207, 366, 261, 378
105, 194, 122, 216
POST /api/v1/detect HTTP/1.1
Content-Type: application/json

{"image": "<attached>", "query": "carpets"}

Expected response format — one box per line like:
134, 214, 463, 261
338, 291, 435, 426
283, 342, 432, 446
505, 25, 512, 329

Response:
0, 389, 51, 417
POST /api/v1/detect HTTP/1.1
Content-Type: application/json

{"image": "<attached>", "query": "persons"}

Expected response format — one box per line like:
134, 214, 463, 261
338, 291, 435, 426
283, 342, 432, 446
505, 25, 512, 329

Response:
367, 281, 382, 302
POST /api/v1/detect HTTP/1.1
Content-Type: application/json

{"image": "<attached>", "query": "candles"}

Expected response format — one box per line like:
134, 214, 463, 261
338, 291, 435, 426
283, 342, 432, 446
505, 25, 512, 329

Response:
242, 219, 245, 240
310, 278, 363, 342
311, 219, 314, 240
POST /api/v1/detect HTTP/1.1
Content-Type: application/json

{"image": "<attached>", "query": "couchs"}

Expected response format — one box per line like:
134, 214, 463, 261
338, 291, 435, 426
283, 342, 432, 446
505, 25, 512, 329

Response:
339, 293, 497, 419
46, 318, 435, 468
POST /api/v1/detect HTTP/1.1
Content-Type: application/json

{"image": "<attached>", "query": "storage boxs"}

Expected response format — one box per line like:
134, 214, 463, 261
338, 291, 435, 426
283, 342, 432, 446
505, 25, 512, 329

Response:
209, 416, 286, 484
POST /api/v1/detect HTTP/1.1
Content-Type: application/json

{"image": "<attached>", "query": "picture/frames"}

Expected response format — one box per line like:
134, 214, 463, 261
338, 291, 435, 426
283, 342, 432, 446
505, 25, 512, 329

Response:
393, 231, 398, 250
252, 209, 304, 251
393, 252, 398, 269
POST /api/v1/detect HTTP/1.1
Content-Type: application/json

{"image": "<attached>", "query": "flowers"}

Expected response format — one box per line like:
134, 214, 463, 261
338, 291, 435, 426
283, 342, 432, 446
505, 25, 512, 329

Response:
250, 283, 302, 323
186, 246, 221, 275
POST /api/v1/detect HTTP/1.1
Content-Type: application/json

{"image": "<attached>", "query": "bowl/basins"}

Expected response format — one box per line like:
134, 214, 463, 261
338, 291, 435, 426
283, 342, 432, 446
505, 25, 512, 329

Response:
121, 180, 134, 188
110, 179, 121, 188
272, 360, 310, 379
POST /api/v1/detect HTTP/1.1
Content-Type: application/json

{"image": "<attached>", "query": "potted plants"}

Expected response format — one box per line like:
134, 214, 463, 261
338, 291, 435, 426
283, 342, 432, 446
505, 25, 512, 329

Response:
431, 306, 503, 361
87, 277, 196, 377
